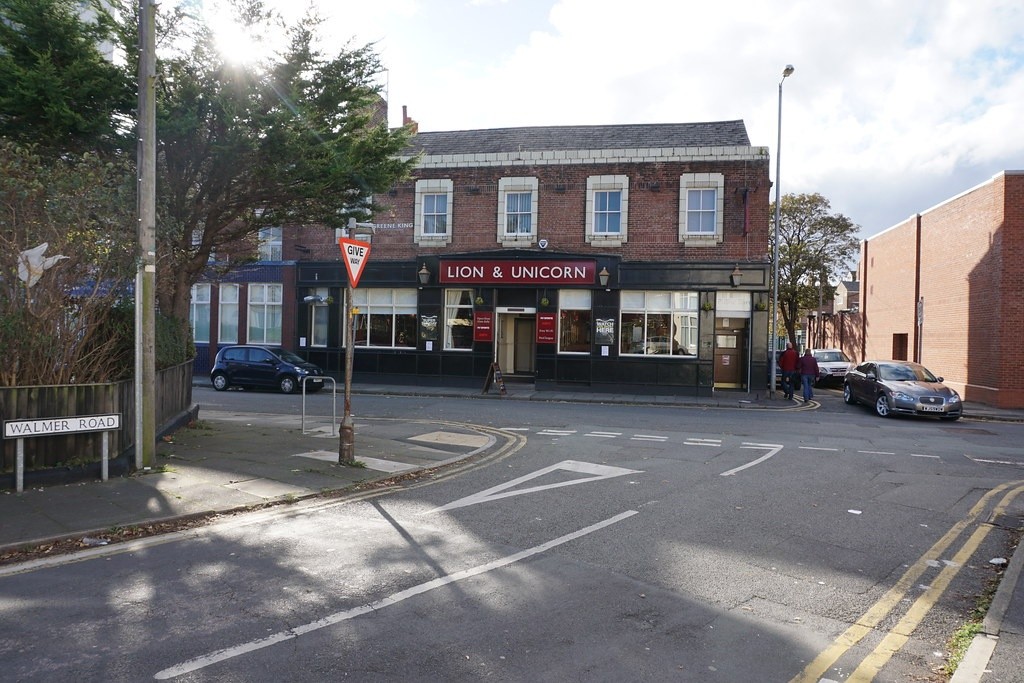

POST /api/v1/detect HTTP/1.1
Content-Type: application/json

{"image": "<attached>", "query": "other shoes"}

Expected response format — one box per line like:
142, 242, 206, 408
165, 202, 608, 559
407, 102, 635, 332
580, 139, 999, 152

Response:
784, 392, 793, 400
805, 397, 813, 403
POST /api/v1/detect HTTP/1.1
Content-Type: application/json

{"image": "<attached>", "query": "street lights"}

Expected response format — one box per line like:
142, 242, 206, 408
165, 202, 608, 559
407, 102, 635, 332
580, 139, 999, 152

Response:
770, 65, 794, 401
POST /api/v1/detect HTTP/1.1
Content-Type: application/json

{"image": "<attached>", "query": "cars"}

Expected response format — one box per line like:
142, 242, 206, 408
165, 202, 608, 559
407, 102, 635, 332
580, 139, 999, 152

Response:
211, 345, 324, 394
768, 349, 963, 421
634, 336, 688, 355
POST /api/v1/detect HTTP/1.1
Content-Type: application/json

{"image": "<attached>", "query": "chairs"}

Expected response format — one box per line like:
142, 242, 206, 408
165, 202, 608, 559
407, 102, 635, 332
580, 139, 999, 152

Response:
828, 355, 838, 359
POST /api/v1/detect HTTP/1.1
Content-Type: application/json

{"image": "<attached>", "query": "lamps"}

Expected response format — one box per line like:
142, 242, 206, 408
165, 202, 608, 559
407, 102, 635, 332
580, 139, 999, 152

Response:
731, 262, 743, 286
556, 188, 566, 193
651, 186, 661, 192
418, 262, 430, 284
389, 190, 396, 195
599, 266, 610, 285
472, 188, 479, 193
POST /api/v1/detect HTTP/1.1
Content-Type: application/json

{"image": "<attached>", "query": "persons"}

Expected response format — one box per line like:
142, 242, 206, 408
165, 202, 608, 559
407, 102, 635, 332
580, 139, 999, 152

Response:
778, 342, 798, 400
796, 349, 819, 402
